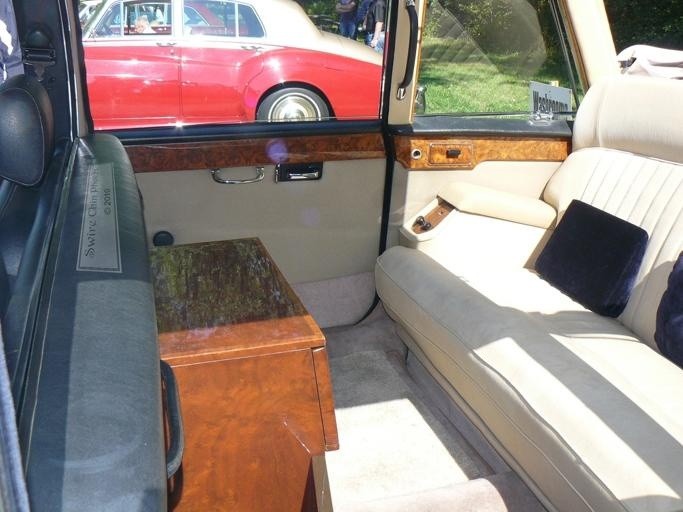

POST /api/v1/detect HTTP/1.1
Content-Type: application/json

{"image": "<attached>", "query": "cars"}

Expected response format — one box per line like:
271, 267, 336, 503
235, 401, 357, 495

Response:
77, 0, 383, 131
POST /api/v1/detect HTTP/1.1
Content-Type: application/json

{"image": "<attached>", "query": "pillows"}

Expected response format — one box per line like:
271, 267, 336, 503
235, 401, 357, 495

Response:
534, 199, 649, 317
653, 252, 683, 369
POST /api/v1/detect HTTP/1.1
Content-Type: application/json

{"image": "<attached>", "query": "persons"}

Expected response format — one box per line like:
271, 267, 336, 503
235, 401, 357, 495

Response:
360, 0, 387, 54
149, 4, 162, 27
334, 0, 358, 40
132, 14, 157, 33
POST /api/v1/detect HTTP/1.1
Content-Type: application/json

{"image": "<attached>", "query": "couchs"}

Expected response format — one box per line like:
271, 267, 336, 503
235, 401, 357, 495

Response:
374, 75, 683, 512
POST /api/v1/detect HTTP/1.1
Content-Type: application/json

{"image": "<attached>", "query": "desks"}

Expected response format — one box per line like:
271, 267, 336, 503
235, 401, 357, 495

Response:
146, 236, 340, 512
